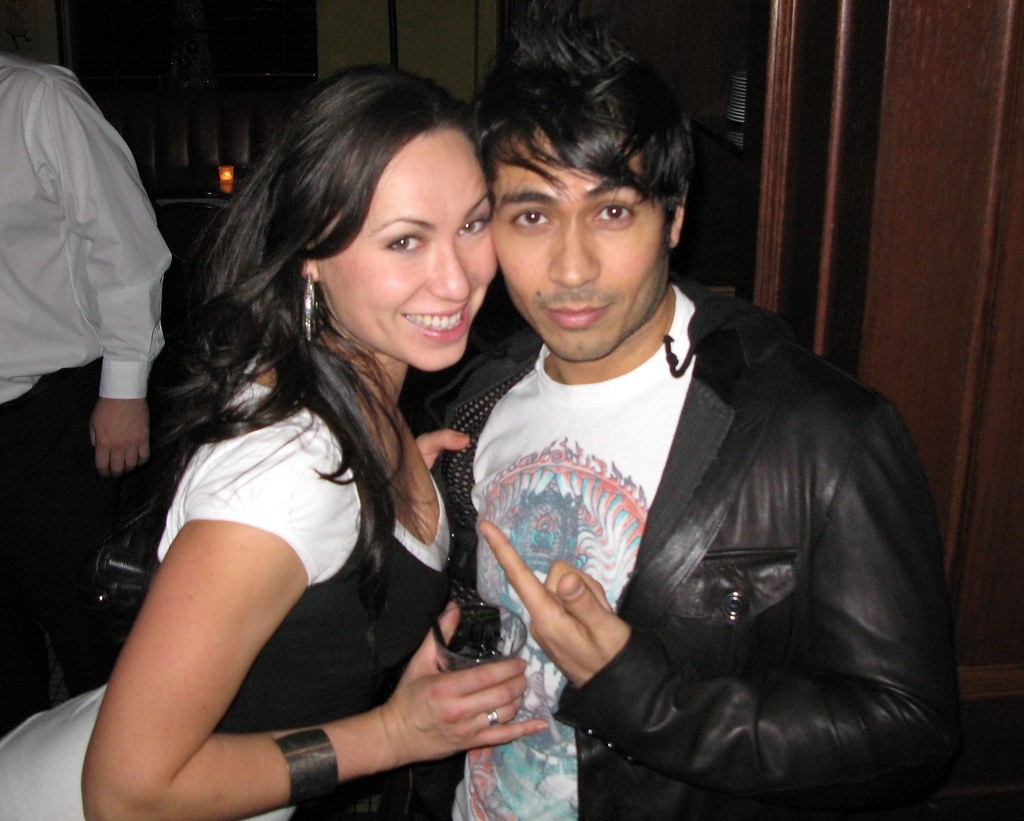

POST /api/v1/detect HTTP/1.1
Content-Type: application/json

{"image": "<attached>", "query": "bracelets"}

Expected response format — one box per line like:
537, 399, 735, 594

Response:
272, 725, 338, 808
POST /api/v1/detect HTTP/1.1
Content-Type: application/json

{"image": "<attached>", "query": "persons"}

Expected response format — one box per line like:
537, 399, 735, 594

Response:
0, 54, 171, 738
81, 1, 961, 821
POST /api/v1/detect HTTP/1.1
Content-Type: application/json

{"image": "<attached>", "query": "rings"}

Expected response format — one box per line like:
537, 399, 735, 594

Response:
487, 710, 498, 725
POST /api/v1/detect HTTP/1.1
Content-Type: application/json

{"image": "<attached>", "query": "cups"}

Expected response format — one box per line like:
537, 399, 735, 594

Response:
432, 601, 528, 673
218, 166, 234, 183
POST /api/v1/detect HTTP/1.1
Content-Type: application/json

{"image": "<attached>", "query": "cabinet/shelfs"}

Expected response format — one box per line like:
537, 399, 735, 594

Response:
64, 0, 320, 206
